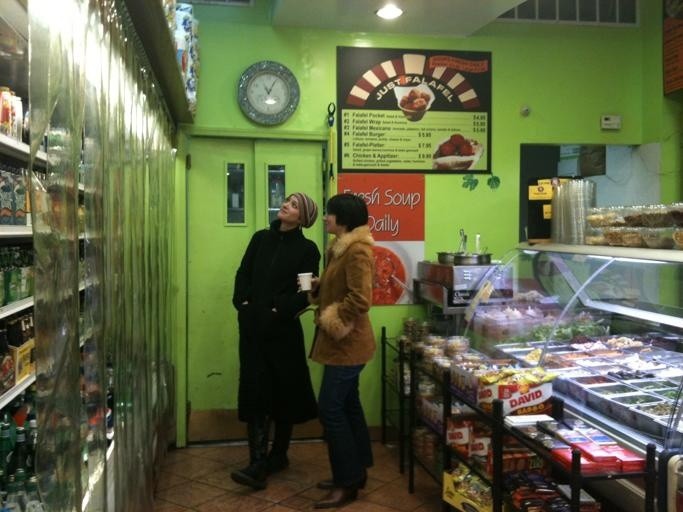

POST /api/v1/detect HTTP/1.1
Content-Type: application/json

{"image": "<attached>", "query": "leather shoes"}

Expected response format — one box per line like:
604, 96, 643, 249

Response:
318, 467, 366, 489
312, 488, 357, 508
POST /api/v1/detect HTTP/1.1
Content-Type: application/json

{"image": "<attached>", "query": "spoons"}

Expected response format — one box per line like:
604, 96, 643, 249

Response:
372, 252, 415, 301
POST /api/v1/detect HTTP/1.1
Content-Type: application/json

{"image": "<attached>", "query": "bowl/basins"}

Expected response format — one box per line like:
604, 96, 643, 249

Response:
432, 146, 483, 169
548, 178, 597, 248
396, 98, 432, 123
584, 203, 683, 248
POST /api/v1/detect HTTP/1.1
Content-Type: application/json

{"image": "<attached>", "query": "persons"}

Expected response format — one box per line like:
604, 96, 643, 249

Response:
230, 191, 320, 490
297, 192, 377, 508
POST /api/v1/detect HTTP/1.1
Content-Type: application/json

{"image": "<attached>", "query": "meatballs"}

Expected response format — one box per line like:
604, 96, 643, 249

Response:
439, 134, 472, 156
400, 88, 429, 110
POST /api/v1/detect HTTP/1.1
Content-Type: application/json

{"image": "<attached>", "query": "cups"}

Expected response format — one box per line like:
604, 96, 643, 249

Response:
296, 271, 313, 292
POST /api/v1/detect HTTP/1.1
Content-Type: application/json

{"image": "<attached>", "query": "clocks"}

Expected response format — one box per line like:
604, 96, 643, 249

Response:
238, 61, 300, 126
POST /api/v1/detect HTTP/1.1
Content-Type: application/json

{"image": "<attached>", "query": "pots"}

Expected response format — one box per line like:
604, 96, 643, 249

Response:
436, 251, 492, 265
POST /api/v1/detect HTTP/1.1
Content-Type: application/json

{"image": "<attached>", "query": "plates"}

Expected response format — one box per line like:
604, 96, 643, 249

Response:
368, 242, 414, 302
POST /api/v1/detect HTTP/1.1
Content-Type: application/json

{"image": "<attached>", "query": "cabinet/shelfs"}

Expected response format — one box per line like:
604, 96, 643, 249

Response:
378, 326, 656, 512
465, 246, 682, 454
0, 131, 164, 512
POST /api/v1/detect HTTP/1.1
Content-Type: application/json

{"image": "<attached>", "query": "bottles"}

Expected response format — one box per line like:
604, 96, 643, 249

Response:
0, 86, 22, 140
0, 412, 60, 512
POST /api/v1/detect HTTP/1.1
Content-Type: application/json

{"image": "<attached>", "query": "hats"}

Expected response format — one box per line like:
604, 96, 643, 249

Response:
293, 192, 318, 228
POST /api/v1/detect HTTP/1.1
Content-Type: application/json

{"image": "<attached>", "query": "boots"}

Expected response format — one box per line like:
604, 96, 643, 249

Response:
230, 423, 267, 491
268, 416, 294, 474
